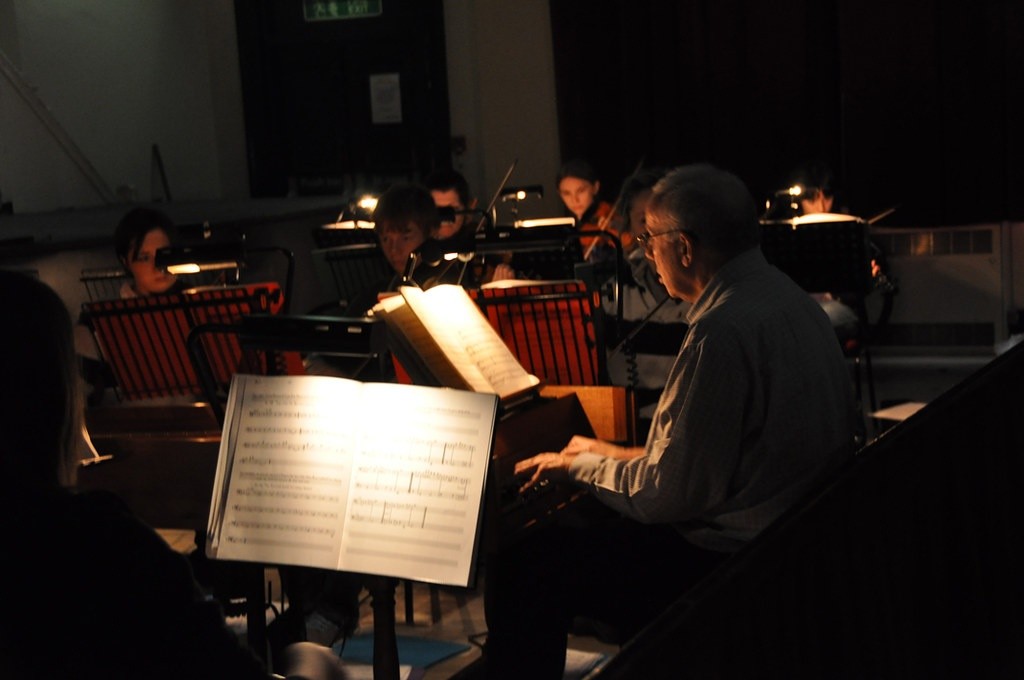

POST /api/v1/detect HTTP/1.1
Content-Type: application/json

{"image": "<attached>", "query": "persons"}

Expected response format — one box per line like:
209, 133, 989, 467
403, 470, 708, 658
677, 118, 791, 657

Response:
449, 163, 855, 680
0, 164, 877, 680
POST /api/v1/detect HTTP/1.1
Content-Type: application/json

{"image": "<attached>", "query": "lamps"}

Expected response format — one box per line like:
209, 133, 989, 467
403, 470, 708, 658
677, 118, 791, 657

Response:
335, 175, 380, 222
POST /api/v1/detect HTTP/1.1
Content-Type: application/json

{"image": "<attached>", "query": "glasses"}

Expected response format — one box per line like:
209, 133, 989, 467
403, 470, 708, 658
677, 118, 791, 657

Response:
636, 228, 701, 246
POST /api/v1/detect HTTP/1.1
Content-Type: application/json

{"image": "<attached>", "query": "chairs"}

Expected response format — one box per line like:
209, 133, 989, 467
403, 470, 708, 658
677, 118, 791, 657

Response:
761, 218, 878, 432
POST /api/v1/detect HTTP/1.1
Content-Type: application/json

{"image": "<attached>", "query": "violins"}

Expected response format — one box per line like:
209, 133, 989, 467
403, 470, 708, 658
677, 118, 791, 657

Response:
579, 200, 635, 251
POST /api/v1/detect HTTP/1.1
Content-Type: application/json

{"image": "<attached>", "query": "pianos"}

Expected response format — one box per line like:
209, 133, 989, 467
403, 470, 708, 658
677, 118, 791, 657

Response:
476, 392, 599, 575
